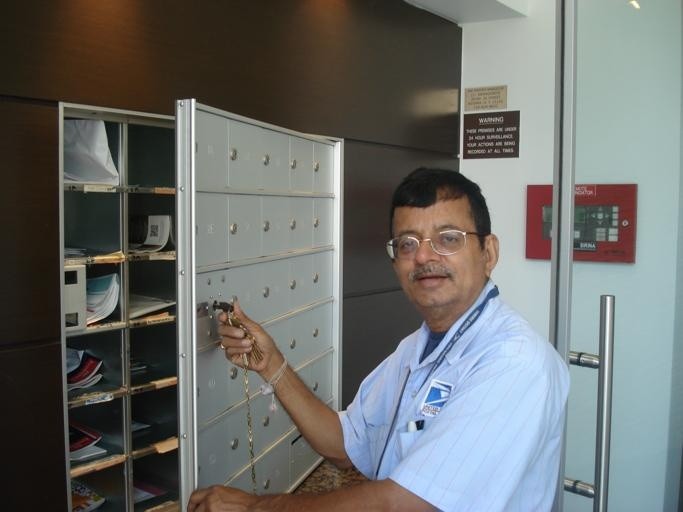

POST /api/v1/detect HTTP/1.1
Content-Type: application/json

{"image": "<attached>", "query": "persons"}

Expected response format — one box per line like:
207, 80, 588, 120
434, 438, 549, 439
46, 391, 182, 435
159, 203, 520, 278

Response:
184, 165, 572, 512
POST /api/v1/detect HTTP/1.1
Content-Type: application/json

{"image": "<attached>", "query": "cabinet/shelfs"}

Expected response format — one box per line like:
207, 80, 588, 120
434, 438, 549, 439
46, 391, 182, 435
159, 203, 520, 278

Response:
59, 104, 343, 512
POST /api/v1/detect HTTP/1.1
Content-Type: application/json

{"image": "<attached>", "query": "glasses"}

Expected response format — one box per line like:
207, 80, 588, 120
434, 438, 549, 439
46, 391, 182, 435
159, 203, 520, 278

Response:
385, 230, 480, 260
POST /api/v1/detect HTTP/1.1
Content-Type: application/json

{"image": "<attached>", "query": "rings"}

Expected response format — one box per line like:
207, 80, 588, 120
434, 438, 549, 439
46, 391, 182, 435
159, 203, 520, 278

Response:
219, 342, 225, 350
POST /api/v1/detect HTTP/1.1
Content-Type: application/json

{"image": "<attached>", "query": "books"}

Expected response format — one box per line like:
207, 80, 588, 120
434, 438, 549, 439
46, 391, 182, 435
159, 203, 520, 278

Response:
86, 272, 119, 325
70, 445, 107, 461
128, 293, 176, 320
68, 373, 103, 392
67, 349, 103, 385
129, 357, 147, 371
70, 479, 105, 511
133, 215, 172, 254
133, 477, 167, 504
68, 417, 101, 454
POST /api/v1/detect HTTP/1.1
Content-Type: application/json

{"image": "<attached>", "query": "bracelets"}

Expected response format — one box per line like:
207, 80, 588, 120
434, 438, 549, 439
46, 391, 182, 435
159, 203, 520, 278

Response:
261, 358, 288, 412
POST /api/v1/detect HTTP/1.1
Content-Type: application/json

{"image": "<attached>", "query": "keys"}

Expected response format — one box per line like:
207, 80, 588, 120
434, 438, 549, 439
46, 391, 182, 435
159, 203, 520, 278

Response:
211, 297, 235, 313
223, 315, 264, 366
